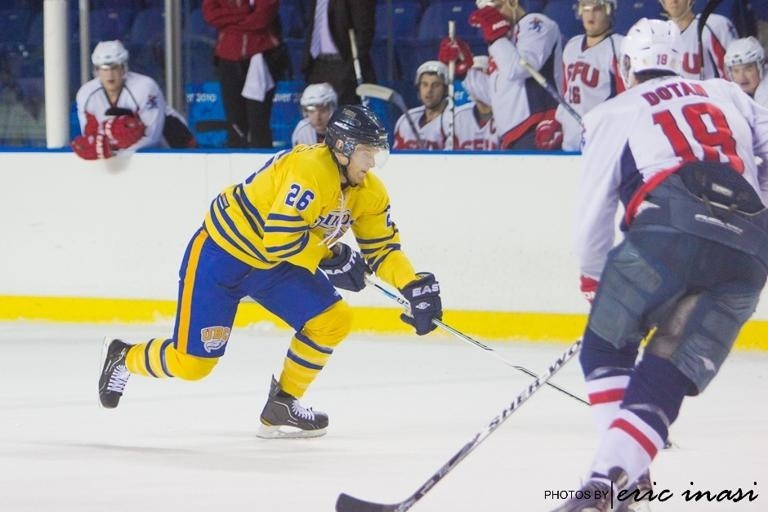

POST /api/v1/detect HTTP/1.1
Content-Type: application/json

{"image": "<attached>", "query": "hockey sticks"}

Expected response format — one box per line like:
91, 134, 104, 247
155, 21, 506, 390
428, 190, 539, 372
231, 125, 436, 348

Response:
105, 108, 136, 118
357, 83, 424, 150
335, 334, 583, 512
195, 118, 254, 148
697, 1, 721, 78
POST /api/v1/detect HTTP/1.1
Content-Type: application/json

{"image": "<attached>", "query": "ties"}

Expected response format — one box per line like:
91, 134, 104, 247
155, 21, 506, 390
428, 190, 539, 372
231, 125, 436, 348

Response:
310, 0, 325, 59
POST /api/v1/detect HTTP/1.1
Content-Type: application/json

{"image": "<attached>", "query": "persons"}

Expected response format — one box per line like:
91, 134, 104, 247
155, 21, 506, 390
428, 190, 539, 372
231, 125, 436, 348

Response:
290, 82, 340, 147
545, 14, 768, 512
96, 102, 443, 431
68, 38, 197, 161
723, 35, 768, 108
657, 0, 739, 81
201, 1, 282, 149
553, 0, 628, 152
298, 0, 376, 107
390, 0, 566, 152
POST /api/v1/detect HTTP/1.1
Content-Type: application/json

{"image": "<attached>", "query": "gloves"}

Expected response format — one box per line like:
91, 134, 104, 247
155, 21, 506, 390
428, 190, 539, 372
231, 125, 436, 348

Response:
101, 117, 144, 148
319, 243, 373, 291
71, 134, 112, 159
469, 6, 509, 41
399, 272, 442, 335
536, 120, 562, 150
439, 37, 473, 73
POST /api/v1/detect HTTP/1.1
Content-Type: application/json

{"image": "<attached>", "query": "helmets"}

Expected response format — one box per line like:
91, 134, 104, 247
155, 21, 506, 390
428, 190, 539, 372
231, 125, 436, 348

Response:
300, 83, 336, 116
92, 40, 128, 69
476, 0, 518, 11
620, 17, 682, 90
724, 37, 764, 82
415, 60, 450, 85
325, 104, 389, 165
577, 0, 615, 18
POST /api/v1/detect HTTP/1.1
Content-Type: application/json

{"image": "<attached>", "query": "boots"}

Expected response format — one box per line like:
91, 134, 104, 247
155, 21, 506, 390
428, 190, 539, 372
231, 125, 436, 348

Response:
616, 469, 651, 507
100, 340, 135, 408
552, 466, 627, 511
260, 376, 328, 429
